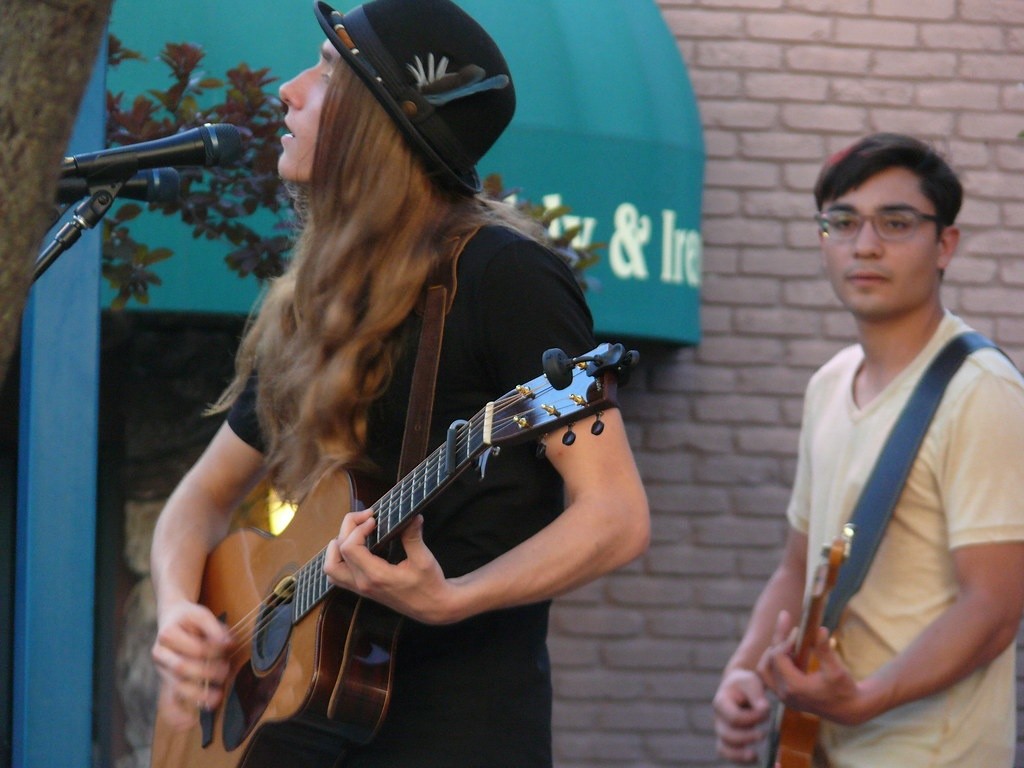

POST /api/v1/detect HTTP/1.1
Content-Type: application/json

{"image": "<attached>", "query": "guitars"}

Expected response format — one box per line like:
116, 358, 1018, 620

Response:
771, 522, 886, 767
151, 338, 641, 767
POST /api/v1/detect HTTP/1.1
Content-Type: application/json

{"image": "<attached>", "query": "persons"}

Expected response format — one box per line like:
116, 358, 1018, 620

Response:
150, 1, 652, 768
711, 133, 1024, 768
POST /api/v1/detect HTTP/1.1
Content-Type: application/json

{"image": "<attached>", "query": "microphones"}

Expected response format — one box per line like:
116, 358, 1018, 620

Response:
61, 122, 245, 180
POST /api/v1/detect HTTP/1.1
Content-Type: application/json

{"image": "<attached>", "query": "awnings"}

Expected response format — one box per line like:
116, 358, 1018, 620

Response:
100, 0, 704, 353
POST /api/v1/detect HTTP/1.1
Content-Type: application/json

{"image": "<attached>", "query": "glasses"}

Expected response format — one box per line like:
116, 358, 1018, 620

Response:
816, 205, 938, 242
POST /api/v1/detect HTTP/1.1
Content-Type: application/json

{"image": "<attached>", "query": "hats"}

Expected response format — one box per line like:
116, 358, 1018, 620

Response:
312, 0, 516, 194
